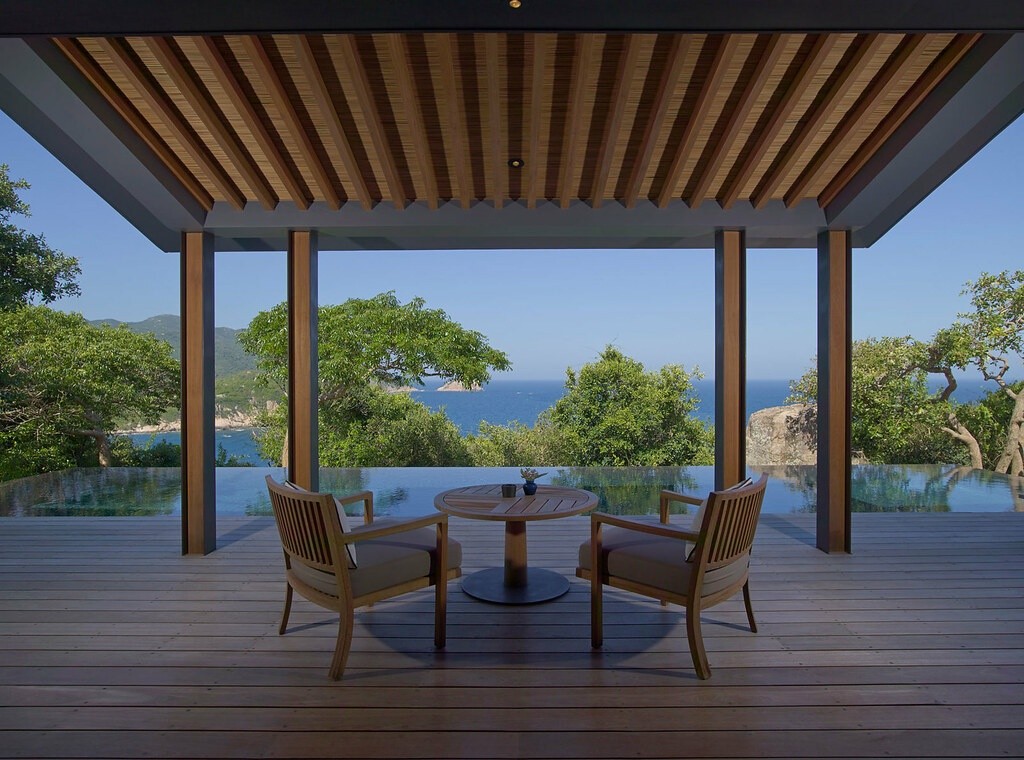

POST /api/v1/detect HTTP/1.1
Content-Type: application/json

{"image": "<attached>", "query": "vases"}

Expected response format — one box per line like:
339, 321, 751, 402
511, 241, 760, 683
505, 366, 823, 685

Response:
523, 481, 539, 495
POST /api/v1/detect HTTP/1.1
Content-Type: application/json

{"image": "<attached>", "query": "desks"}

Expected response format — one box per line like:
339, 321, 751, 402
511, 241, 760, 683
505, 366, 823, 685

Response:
435, 484, 599, 605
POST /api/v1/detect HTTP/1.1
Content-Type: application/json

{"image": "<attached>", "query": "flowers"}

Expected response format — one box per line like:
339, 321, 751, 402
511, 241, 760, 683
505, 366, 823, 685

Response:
519, 469, 545, 482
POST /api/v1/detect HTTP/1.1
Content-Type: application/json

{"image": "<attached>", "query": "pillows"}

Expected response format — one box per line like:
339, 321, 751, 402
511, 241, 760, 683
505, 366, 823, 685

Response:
684, 476, 753, 562
285, 481, 358, 568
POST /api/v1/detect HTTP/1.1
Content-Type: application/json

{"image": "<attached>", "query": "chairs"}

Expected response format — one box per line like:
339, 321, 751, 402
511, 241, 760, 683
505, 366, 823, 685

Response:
266, 471, 462, 679
575, 470, 768, 681
